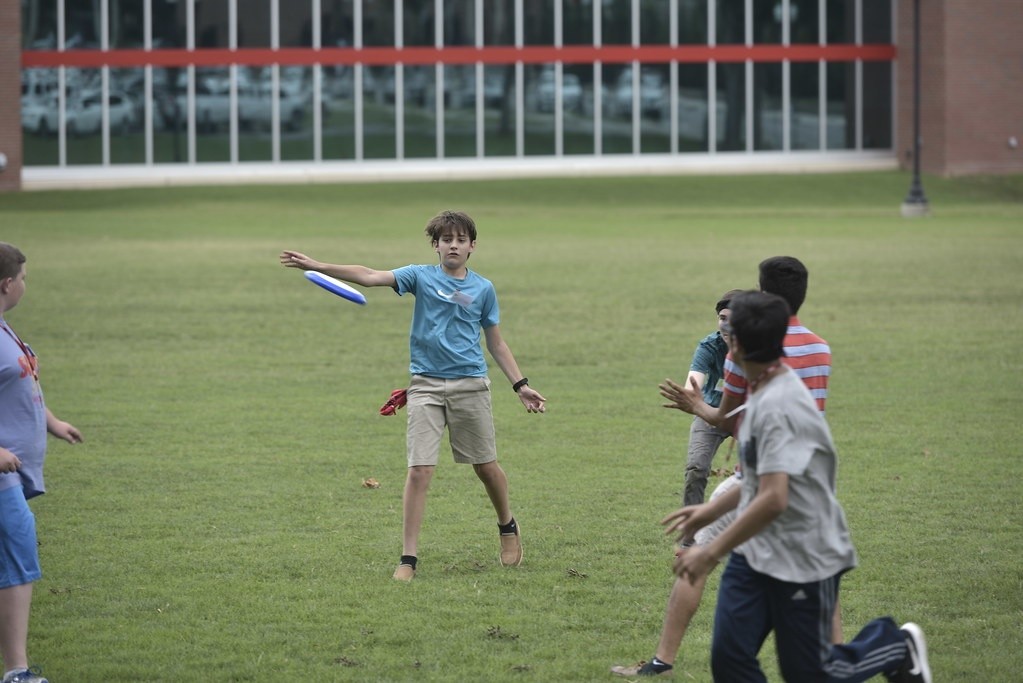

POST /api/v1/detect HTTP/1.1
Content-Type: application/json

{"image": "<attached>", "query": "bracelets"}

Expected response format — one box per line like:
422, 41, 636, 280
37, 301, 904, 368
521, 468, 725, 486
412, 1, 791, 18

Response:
513, 377, 529, 392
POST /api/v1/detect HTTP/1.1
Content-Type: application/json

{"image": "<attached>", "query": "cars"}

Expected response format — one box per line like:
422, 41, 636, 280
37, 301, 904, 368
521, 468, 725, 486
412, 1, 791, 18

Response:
17, 62, 670, 143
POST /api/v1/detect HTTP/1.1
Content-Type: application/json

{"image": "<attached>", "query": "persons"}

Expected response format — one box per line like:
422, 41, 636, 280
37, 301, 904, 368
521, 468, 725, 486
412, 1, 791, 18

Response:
680, 289, 739, 547
661, 287, 935, 683
608, 255, 832, 676
280, 210, 546, 583
0, 242, 84, 683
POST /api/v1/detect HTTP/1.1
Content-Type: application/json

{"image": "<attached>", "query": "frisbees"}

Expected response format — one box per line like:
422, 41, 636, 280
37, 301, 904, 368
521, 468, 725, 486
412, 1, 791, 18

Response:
304, 267, 368, 306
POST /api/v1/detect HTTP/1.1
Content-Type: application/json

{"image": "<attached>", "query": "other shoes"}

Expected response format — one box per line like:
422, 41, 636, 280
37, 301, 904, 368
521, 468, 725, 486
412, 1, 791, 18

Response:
676, 536, 693, 555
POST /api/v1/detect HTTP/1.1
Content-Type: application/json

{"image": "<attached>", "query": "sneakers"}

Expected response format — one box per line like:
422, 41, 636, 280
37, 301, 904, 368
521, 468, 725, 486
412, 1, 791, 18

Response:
4, 665, 47, 683
611, 657, 671, 677
500, 519, 522, 569
883, 622, 931, 683
392, 563, 414, 583
